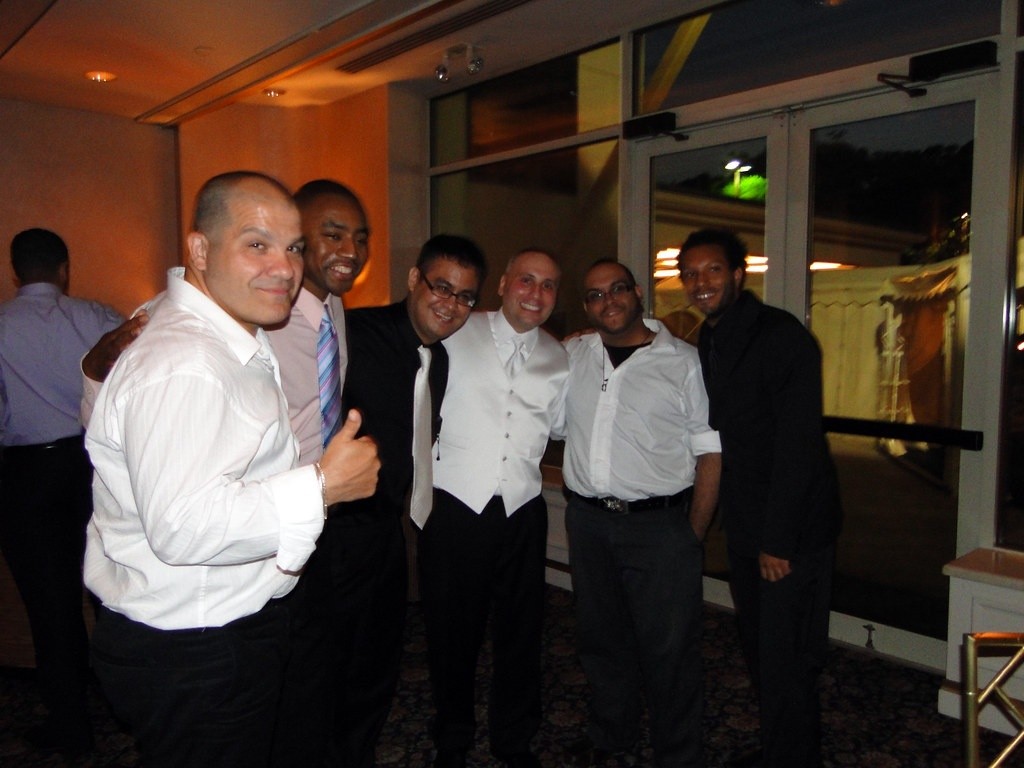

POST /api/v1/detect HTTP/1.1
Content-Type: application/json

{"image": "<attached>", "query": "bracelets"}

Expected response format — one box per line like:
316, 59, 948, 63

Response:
314, 464, 328, 520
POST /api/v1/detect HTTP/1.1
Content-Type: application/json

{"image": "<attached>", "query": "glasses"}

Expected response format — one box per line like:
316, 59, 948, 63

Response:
583, 280, 634, 305
421, 272, 479, 308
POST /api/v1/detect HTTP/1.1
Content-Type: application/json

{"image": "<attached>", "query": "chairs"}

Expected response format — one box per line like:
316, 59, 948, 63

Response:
958, 631, 1024, 768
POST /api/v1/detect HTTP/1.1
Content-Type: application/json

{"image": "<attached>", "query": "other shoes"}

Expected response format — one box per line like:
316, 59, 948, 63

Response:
434, 749, 467, 768
489, 746, 541, 768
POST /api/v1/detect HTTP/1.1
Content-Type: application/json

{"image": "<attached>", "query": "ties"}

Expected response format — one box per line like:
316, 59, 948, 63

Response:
317, 304, 342, 453
409, 345, 433, 530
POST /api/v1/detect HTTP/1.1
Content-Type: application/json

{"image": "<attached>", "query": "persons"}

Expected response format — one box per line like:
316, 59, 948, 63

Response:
0, 228, 130, 757
78, 171, 380, 768
291, 227, 830, 768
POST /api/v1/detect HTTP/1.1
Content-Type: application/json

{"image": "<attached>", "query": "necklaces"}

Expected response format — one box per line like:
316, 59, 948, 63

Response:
601, 327, 652, 392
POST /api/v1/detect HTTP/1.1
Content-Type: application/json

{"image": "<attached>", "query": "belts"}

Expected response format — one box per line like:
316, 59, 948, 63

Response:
3, 435, 85, 452
575, 485, 698, 516
